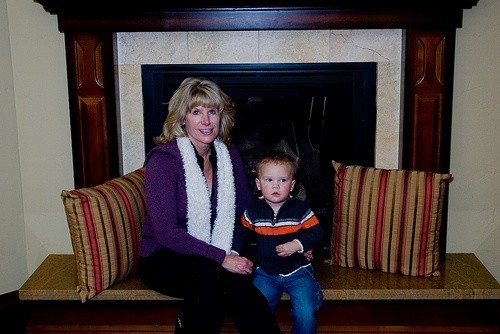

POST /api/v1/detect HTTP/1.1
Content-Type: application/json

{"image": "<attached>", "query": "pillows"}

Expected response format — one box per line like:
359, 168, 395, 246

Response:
326, 160, 451, 280
62, 163, 150, 304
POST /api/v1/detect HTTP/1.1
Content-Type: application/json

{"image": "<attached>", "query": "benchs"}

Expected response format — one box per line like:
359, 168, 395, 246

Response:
18, 254, 499, 301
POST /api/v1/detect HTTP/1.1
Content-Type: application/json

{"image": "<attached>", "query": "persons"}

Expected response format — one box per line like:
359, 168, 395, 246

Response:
135, 77, 284, 334
228, 152, 328, 334
